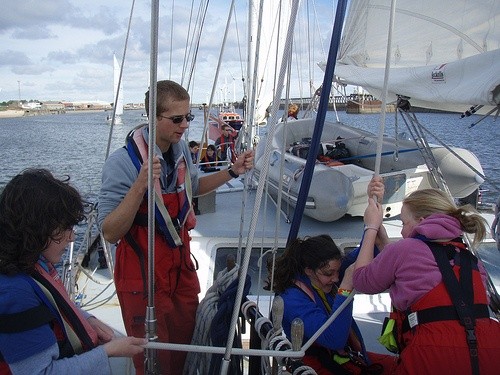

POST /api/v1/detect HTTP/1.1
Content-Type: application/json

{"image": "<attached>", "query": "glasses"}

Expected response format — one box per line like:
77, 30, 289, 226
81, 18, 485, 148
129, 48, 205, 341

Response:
159, 113, 195, 124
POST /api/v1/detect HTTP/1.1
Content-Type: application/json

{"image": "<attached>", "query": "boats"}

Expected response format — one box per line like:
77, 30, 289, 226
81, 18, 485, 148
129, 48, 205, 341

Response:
255, 118, 486, 224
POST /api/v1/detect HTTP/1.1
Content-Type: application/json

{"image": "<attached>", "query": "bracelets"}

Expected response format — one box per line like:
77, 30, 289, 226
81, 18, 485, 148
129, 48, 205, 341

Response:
227, 167, 239, 178
364, 224, 378, 231
338, 288, 351, 296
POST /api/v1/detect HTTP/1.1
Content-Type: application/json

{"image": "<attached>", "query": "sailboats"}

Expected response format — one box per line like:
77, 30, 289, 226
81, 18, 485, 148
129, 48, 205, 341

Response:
105, 51, 125, 125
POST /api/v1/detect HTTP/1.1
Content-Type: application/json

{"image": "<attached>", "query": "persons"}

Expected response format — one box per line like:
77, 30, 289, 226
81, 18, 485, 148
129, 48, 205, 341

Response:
188, 128, 237, 172
0, 168, 148, 375
353, 189, 500, 375
97, 80, 254, 375
262, 174, 385, 375
